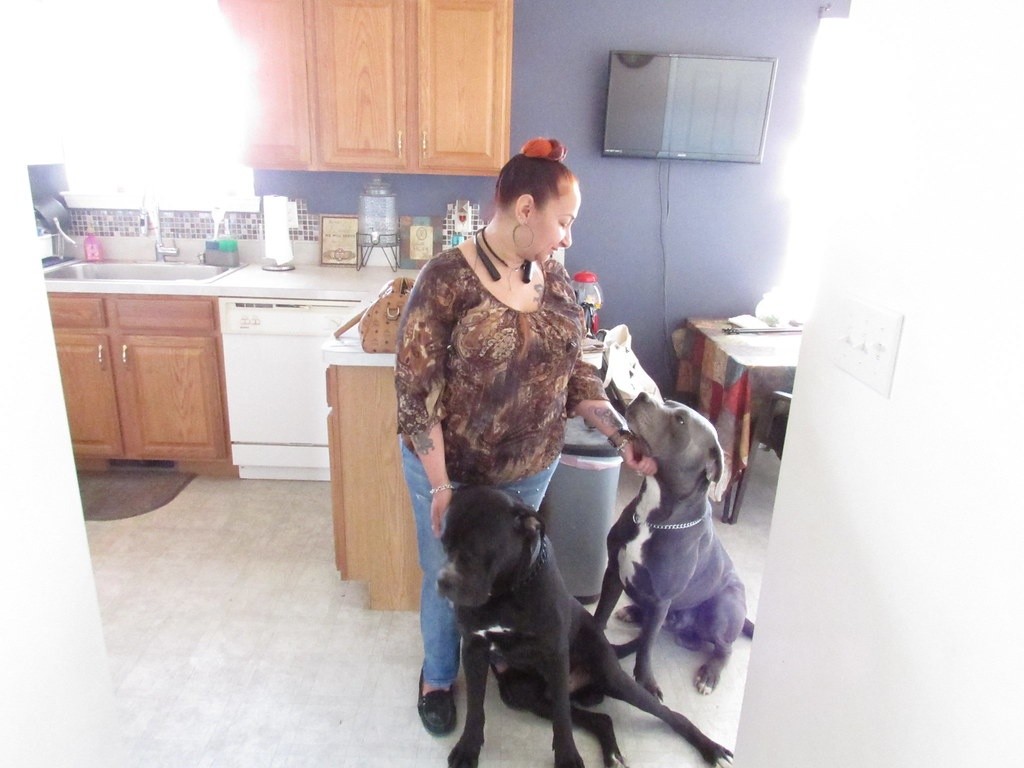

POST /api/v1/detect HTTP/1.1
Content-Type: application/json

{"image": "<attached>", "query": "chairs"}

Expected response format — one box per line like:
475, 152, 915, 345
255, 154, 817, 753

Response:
729, 389, 794, 525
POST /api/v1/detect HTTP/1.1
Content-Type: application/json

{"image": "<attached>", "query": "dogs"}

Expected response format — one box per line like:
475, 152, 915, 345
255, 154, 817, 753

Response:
594, 393, 754, 702
436, 483, 737, 768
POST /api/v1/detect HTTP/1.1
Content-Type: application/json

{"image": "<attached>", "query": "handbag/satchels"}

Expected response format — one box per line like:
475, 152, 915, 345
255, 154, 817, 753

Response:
332, 274, 419, 354
601, 323, 666, 407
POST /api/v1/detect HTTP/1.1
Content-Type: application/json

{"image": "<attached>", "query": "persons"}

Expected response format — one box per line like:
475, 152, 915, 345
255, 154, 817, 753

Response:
395, 139, 658, 734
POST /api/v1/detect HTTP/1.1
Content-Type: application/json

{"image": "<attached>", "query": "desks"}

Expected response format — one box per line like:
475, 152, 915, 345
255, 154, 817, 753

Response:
673, 315, 804, 526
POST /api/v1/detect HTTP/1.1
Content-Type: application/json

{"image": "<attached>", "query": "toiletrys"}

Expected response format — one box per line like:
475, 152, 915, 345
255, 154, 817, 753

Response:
83, 227, 105, 263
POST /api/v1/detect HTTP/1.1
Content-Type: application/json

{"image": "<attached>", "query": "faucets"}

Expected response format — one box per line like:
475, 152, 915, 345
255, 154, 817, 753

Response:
141, 190, 179, 263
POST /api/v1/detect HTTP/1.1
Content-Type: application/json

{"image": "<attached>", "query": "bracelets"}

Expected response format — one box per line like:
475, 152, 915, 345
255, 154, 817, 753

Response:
616, 438, 628, 451
430, 484, 454, 494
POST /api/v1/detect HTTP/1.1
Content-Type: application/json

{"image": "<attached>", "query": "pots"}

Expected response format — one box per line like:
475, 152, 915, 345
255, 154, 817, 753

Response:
33, 194, 78, 249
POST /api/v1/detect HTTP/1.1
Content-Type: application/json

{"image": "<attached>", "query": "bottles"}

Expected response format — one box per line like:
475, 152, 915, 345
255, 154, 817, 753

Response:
570, 269, 602, 336
358, 175, 399, 248
83, 232, 104, 263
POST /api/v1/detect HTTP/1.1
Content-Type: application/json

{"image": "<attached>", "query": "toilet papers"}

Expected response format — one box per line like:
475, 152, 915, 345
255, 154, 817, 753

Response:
262, 195, 295, 267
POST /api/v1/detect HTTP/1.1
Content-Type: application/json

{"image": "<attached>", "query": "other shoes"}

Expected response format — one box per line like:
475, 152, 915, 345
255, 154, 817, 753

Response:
491, 656, 522, 708
419, 663, 457, 734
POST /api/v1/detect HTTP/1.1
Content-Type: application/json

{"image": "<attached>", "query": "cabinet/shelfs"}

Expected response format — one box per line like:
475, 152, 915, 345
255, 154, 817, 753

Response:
43, 293, 234, 476
325, 366, 423, 613
302, 0, 512, 178
214, 1, 302, 172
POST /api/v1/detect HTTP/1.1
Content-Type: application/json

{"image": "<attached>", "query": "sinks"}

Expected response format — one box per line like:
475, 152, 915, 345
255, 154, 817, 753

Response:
42, 259, 250, 284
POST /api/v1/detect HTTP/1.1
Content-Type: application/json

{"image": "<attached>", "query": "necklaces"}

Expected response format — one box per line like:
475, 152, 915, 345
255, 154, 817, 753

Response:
482, 226, 526, 271
